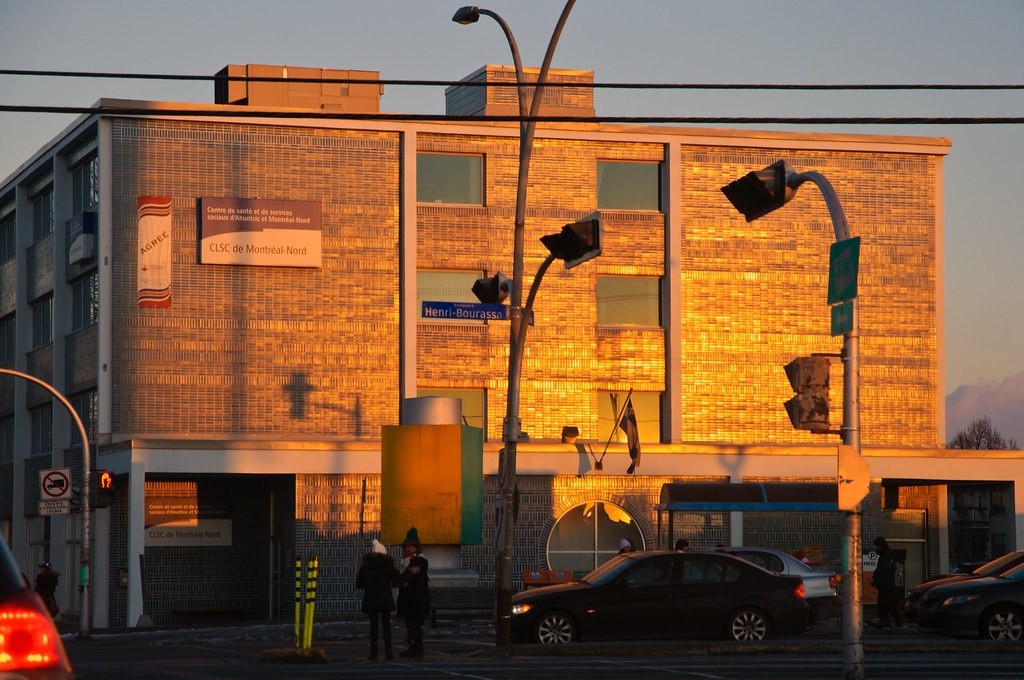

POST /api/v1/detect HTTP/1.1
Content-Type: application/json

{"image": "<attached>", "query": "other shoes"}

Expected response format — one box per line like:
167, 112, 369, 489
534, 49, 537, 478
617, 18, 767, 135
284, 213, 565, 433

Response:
875, 620, 902, 630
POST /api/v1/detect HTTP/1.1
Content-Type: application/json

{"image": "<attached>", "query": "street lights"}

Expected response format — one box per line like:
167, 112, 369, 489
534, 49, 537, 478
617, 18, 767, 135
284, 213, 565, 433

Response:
451, 0, 577, 643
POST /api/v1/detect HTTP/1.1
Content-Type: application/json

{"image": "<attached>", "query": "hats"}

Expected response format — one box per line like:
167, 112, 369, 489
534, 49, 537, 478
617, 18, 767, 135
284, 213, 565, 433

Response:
619, 538, 631, 550
404, 528, 420, 546
38, 561, 52, 568
874, 537, 887, 546
794, 551, 805, 560
373, 539, 386, 555
676, 540, 689, 548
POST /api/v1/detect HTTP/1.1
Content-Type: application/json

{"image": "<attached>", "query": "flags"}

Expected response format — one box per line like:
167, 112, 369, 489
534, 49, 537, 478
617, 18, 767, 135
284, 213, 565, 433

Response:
618, 399, 639, 473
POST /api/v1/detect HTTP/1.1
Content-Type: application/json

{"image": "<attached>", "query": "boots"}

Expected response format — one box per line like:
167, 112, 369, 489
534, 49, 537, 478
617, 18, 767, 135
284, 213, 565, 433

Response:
400, 630, 425, 658
368, 617, 378, 660
382, 617, 394, 660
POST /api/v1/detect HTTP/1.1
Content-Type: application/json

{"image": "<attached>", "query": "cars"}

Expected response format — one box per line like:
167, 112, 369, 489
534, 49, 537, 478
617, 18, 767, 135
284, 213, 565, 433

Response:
509, 550, 812, 643
920, 561, 1024, 640
1, 533, 75, 680
713, 546, 838, 617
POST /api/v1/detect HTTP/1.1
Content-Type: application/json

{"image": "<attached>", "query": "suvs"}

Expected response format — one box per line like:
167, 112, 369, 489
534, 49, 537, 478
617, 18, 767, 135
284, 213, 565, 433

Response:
904, 550, 1024, 624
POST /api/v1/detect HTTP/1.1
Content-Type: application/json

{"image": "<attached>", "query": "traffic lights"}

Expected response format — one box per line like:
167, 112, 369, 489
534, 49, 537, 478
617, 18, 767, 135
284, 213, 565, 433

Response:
720, 158, 801, 224
539, 210, 603, 270
471, 271, 509, 304
95, 470, 115, 509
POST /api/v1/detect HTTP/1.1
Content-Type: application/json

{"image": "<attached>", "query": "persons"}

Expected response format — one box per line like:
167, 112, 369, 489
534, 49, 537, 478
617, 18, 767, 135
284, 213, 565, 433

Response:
794, 552, 821, 627
34, 562, 61, 606
619, 540, 633, 555
395, 526, 432, 658
675, 539, 690, 551
870, 537, 907, 630
357, 539, 400, 661
